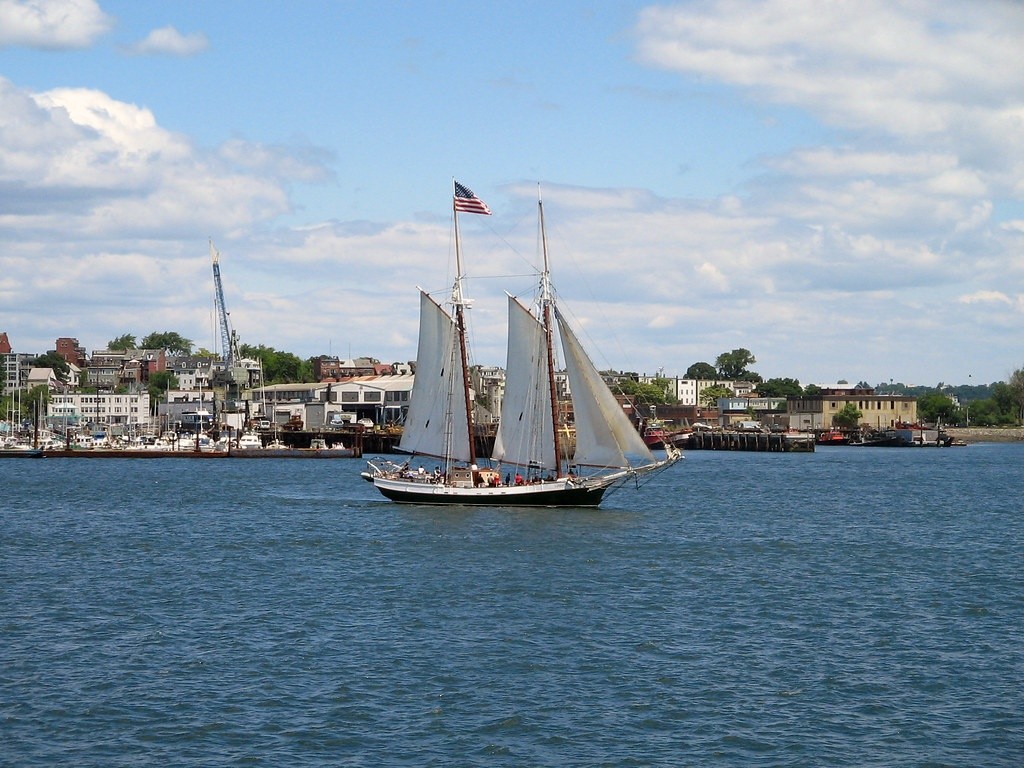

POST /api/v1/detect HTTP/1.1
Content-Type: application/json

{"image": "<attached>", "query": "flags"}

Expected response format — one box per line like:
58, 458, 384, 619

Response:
452, 178, 493, 216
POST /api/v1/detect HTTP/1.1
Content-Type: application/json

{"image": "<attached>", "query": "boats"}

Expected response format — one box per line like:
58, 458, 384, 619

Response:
281, 414, 304, 431
643, 404, 672, 449
331, 442, 346, 450
310, 437, 328, 450
818, 430, 851, 445
330, 413, 344, 428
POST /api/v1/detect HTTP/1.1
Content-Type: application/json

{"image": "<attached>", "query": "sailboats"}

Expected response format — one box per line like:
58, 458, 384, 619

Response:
0, 355, 289, 450
359, 174, 686, 511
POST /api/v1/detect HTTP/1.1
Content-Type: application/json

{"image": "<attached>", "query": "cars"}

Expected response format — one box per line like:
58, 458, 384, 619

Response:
359, 417, 375, 428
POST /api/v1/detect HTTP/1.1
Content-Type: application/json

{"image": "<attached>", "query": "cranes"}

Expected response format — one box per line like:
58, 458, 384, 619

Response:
212, 255, 250, 387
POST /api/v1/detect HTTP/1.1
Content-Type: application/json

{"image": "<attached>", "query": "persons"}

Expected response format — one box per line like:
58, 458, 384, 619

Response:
397, 462, 582, 487
